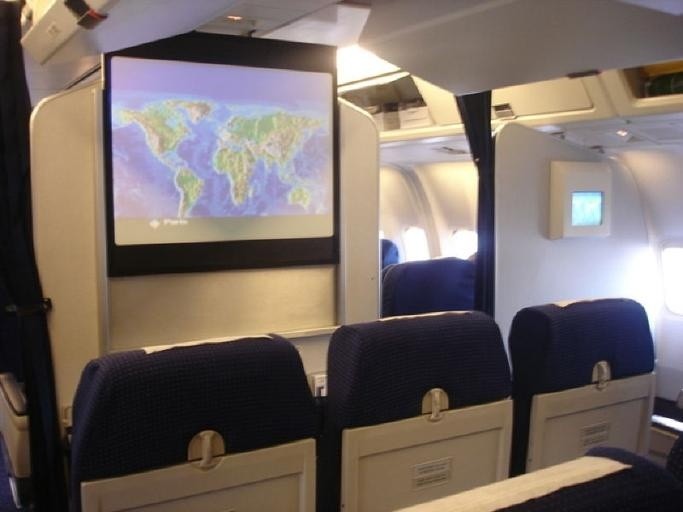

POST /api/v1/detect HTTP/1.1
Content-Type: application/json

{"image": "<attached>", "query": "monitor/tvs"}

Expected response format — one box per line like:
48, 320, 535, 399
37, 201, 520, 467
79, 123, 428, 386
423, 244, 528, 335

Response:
104, 32, 340, 279
571, 191, 604, 229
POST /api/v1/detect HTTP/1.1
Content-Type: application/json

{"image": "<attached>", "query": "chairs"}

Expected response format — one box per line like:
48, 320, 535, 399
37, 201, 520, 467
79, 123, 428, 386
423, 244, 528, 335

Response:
383, 239, 474, 316
68, 297, 680, 510
